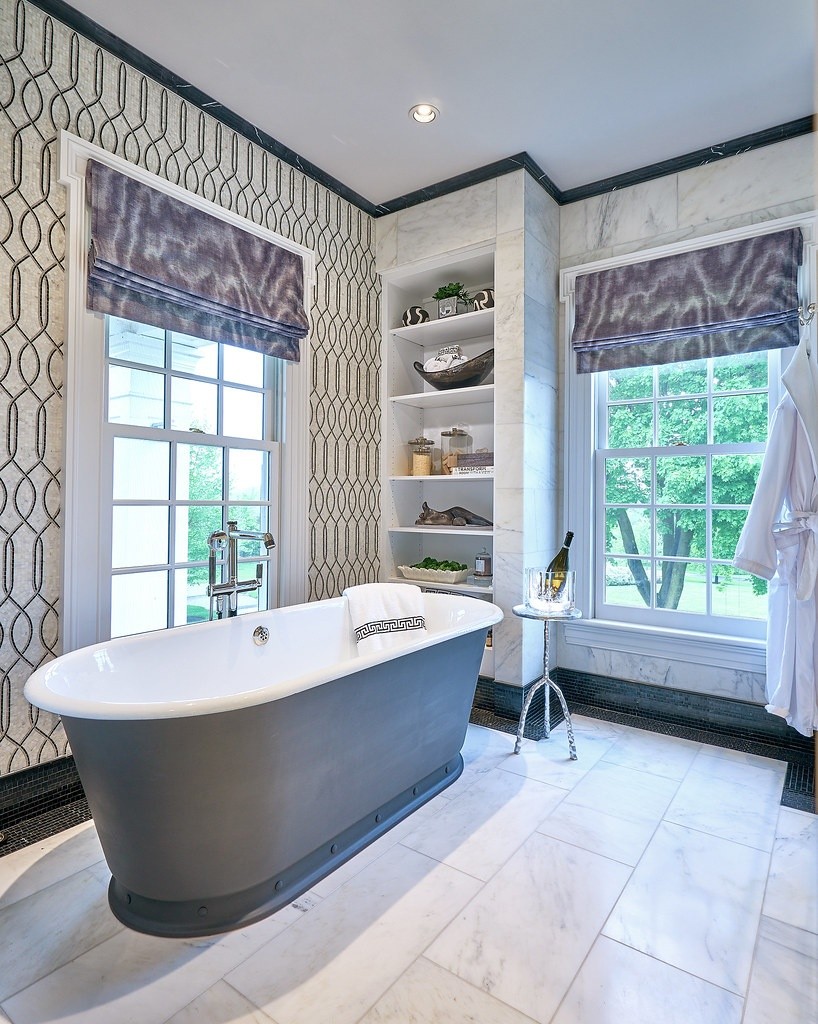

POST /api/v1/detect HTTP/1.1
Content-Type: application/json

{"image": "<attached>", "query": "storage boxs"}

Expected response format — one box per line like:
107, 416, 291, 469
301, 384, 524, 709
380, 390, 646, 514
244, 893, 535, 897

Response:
452, 452, 495, 476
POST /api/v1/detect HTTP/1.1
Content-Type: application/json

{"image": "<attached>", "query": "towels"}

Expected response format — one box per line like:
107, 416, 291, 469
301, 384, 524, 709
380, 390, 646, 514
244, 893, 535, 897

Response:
421, 344, 472, 374
340, 582, 430, 657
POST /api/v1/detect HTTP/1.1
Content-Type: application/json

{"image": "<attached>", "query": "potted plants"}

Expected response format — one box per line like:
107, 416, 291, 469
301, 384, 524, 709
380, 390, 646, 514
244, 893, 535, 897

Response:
432, 281, 471, 319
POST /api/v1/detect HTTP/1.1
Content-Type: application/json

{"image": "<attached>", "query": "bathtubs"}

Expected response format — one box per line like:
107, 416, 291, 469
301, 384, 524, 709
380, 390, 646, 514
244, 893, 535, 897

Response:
23, 593, 504, 940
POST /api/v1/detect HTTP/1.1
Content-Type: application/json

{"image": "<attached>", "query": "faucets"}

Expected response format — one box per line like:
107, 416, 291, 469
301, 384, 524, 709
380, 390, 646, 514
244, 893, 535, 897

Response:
227, 520, 276, 585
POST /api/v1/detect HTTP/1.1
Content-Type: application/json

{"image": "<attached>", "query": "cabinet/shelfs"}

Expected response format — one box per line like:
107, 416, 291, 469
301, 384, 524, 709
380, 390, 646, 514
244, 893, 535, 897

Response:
377, 244, 497, 677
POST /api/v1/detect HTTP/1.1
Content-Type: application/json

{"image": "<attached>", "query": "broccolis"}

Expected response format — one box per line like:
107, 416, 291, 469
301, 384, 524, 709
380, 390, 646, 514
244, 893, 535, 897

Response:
409, 556, 468, 572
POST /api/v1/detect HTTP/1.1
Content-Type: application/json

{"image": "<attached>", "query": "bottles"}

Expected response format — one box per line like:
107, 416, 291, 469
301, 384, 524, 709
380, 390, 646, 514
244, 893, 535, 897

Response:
440, 427, 469, 475
544, 531, 574, 600
407, 436, 435, 476
474, 547, 491, 576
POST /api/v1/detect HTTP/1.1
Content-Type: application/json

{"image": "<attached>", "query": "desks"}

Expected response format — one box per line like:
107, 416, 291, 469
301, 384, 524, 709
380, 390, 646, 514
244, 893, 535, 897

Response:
512, 604, 583, 760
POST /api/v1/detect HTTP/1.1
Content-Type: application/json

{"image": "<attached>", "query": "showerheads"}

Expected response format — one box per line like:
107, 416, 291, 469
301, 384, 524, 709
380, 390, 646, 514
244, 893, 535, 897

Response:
205, 528, 229, 585
